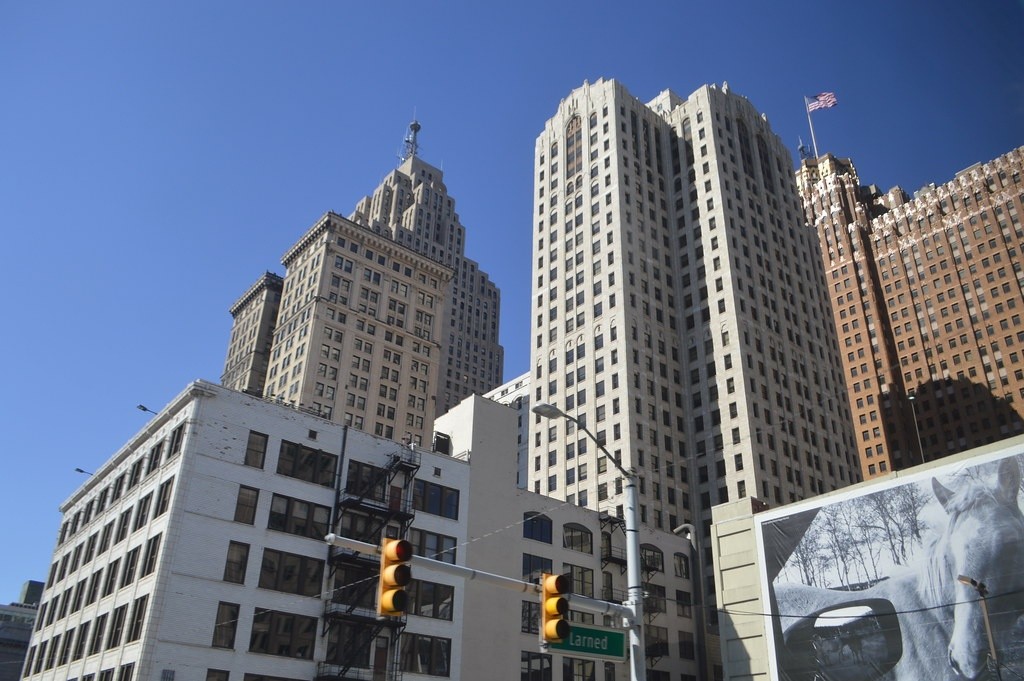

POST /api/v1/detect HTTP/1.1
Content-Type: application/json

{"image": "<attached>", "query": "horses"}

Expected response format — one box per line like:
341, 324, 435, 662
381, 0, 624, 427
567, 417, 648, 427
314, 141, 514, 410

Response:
848, 636, 865, 665
821, 632, 853, 667
768, 456, 1024, 681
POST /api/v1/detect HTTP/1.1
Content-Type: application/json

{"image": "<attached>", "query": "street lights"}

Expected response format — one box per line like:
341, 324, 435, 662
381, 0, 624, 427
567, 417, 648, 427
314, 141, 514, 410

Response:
377, 537, 412, 617
531, 402, 647, 681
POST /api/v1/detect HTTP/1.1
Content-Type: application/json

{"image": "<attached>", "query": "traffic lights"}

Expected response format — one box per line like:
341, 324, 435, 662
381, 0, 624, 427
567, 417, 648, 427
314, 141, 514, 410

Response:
541, 572, 571, 645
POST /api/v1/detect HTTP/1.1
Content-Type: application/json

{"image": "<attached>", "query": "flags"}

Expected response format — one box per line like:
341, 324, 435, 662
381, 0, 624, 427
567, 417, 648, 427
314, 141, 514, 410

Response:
806, 91, 838, 112
